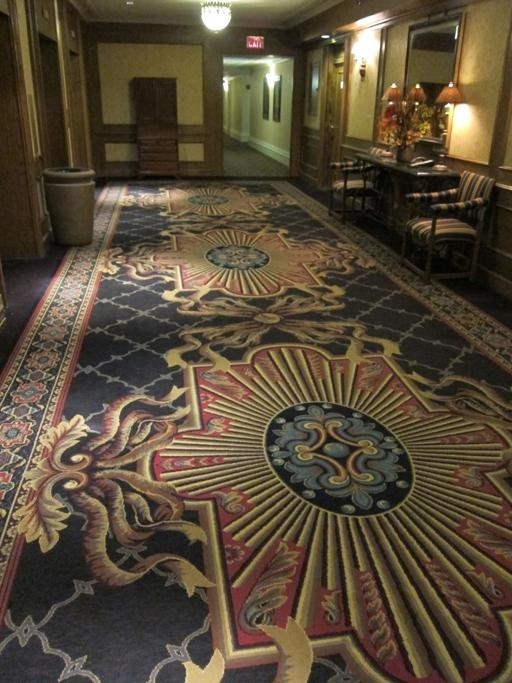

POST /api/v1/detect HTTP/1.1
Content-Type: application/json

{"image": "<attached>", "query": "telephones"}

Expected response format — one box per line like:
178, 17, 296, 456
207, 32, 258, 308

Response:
407, 156, 434, 168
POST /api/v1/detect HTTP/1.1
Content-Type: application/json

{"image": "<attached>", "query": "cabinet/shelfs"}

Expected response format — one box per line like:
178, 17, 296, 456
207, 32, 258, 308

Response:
131, 76, 181, 180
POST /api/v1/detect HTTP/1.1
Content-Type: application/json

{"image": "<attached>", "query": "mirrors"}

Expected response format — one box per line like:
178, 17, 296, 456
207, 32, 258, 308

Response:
402, 10, 466, 149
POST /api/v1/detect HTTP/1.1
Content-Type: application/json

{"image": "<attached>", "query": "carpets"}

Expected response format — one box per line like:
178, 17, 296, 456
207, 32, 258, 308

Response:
0, 180, 512, 682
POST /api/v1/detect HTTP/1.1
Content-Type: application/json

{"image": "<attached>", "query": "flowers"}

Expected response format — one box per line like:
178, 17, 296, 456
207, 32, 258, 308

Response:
379, 101, 431, 147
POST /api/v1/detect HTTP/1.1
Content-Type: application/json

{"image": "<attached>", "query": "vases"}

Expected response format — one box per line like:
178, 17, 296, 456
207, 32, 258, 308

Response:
396, 144, 416, 163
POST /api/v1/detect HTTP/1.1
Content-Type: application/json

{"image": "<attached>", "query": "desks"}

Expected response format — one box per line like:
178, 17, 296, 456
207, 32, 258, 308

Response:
357, 153, 461, 243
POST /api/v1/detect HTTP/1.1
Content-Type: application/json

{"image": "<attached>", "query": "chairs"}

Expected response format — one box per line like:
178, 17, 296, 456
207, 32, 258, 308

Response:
327, 146, 394, 225
399, 170, 497, 284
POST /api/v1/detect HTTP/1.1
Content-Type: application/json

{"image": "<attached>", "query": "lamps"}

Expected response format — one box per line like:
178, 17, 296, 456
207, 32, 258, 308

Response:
201, 1, 232, 34
435, 82, 463, 102
381, 83, 401, 102
408, 83, 425, 98
359, 64, 366, 76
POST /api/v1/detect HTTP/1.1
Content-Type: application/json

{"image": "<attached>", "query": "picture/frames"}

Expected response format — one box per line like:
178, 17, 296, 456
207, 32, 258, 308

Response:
262, 74, 281, 123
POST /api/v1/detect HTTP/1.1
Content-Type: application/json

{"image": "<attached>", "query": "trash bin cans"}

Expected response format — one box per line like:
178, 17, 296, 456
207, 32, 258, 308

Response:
42, 168, 96, 246
